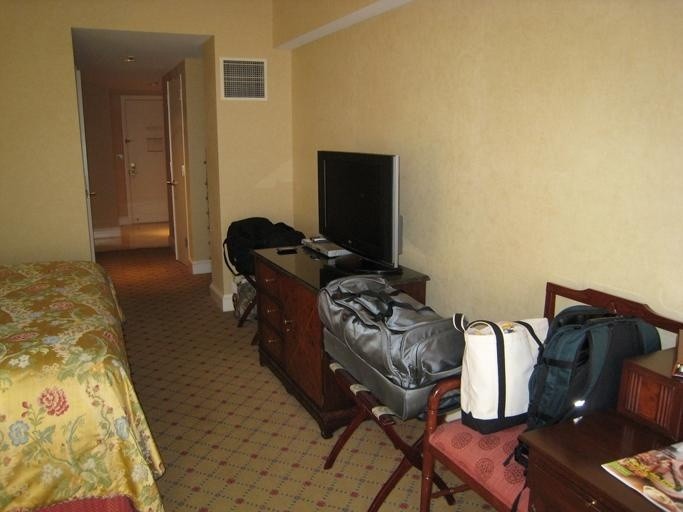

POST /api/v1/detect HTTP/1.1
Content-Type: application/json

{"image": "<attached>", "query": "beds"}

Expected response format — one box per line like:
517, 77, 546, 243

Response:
2, 258, 136, 511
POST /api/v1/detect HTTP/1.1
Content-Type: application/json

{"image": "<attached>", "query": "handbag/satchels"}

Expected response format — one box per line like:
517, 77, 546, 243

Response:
222, 217, 305, 277
317, 273, 549, 435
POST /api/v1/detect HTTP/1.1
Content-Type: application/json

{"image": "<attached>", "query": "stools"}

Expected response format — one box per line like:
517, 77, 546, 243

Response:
233, 266, 258, 328
324, 363, 464, 511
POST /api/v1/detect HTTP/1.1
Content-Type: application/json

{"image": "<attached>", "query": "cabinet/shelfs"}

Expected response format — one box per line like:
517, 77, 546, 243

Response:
518, 407, 674, 511
254, 243, 431, 439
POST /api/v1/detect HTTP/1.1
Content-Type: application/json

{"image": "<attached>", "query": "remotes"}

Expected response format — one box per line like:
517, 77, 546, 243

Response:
277, 247, 296, 253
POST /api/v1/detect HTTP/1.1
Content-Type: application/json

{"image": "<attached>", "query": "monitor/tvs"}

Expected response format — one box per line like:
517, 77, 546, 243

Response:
316, 149, 404, 274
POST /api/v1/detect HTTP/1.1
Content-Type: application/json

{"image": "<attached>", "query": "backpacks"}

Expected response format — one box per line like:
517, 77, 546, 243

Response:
528, 304, 661, 429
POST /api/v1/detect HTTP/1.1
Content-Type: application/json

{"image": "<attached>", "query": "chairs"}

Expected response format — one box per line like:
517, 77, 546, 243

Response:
420, 278, 683, 511
